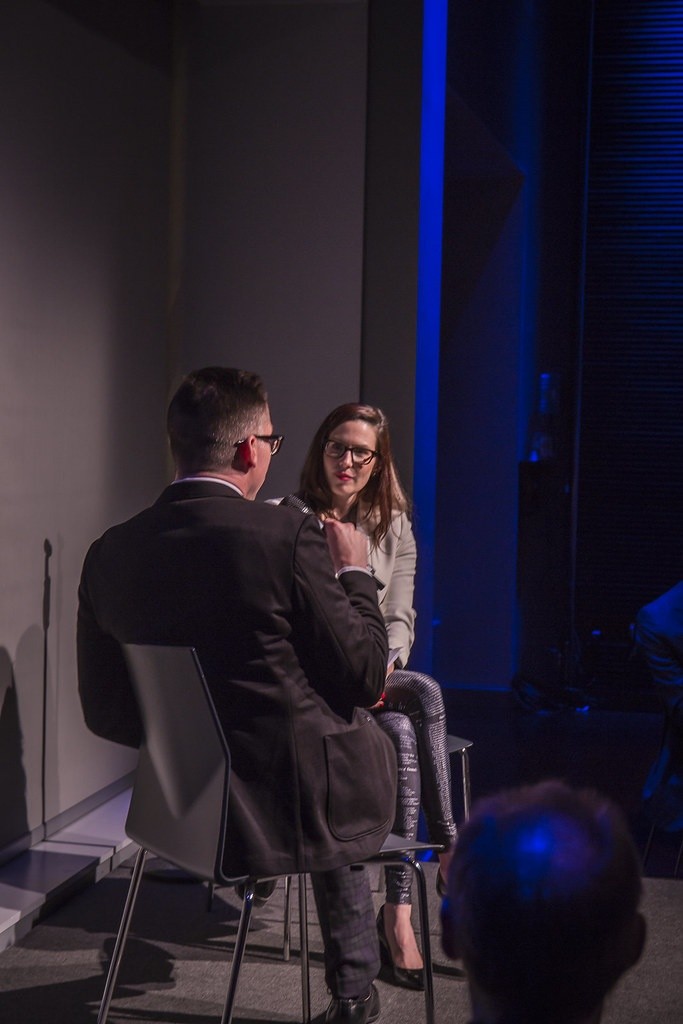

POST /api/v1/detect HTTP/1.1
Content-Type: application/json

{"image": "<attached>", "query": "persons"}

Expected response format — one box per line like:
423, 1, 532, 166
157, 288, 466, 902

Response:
631, 580, 683, 775
261, 403, 457, 991
70, 367, 400, 1024
441, 781, 647, 1024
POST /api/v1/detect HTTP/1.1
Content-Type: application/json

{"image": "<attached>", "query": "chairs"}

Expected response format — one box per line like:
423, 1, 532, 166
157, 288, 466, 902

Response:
96, 640, 446, 1024
206, 734, 475, 961
641, 671, 683, 878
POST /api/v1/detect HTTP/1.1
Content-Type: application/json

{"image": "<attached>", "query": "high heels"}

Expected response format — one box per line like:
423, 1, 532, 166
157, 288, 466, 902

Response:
436, 866, 448, 899
376, 904, 425, 992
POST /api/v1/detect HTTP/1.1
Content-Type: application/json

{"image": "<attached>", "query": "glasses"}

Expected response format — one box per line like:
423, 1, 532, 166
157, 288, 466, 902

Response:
236, 435, 286, 456
324, 439, 379, 465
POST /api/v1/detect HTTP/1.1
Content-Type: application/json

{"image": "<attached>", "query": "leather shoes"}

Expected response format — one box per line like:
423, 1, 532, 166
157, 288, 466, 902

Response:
236, 879, 279, 907
325, 984, 380, 1024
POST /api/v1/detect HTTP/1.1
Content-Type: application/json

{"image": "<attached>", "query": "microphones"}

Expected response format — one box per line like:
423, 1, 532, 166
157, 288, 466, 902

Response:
277, 494, 384, 591
